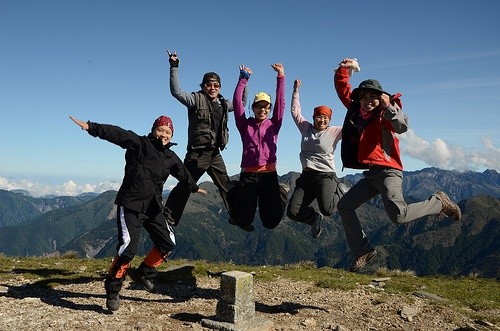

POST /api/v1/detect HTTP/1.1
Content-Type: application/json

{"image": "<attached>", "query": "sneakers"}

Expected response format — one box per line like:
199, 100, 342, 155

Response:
435, 191, 461, 222
350, 250, 377, 269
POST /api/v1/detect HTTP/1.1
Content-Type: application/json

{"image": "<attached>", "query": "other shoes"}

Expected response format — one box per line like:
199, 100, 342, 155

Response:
104, 281, 120, 310
280, 182, 291, 192
310, 212, 323, 238
134, 262, 155, 291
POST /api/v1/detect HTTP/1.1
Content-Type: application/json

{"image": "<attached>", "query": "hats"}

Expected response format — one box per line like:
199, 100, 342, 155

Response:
153, 115, 174, 131
199, 72, 220, 85
313, 106, 332, 119
348, 79, 391, 100
254, 91, 271, 103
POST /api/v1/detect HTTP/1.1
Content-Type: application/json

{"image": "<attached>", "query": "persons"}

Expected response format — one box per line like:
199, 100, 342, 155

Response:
333, 58, 462, 274
165, 49, 256, 232
286, 80, 342, 239
232, 63, 290, 230
69, 115, 207, 311
